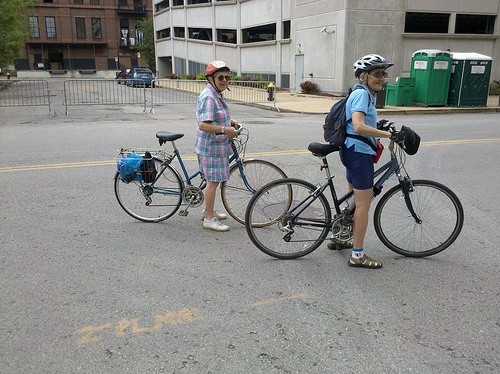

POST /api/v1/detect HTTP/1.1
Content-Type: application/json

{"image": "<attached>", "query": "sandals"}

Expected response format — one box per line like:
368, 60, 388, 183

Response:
327, 237, 352, 249
349, 254, 382, 268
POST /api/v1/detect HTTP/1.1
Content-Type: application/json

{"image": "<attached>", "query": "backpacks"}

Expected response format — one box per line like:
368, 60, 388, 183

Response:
323, 86, 370, 145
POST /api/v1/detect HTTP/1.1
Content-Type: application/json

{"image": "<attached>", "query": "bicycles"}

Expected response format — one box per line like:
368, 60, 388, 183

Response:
114, 123, 294, 227
245, 124, 465, 259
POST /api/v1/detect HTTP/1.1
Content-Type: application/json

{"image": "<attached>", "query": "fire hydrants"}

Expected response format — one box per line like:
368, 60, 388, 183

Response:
266, 82, 277, 101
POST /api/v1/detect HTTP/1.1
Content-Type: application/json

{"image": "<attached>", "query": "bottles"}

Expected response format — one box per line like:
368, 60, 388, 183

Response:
344, 195, 356, 211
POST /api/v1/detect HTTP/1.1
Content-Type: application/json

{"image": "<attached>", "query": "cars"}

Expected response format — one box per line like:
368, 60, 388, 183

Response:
116, 69, 156, 87
0, 63, 18, 76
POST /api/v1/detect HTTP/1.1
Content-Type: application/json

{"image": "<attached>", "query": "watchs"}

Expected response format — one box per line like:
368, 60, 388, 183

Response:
221, 126, 225, 134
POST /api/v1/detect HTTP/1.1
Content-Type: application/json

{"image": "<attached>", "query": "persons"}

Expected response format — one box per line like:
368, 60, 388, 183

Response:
327, 54, 405, 269
196, 61, 239, 231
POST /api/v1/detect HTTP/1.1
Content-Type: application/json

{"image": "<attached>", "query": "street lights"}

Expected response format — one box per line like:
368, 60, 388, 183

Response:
119, 25, 129, 54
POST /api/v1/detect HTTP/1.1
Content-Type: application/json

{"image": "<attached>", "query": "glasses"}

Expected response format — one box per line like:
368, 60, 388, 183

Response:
369, 71, 388, 78
212, 75, 230, 82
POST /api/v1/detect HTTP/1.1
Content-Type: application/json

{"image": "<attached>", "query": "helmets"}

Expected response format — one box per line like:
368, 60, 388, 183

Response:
205, 61, 230, 78
353, 54, 395, 77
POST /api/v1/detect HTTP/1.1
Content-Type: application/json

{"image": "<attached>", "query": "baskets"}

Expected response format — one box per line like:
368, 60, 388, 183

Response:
114, 152, 158, 184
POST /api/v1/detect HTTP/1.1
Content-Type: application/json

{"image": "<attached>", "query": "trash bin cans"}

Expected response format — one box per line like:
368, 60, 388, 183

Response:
447, 52, 493, 107
410, 49, 453, 107
385, 77, 416, 107
374, 82, 388, 109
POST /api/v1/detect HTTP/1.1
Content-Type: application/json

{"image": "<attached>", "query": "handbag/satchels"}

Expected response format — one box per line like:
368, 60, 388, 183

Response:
400, 125, 421, 155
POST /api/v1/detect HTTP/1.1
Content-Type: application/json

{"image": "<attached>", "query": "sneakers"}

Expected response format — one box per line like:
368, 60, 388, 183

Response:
203, 217, 229, 232
202, 209, 227, 220
